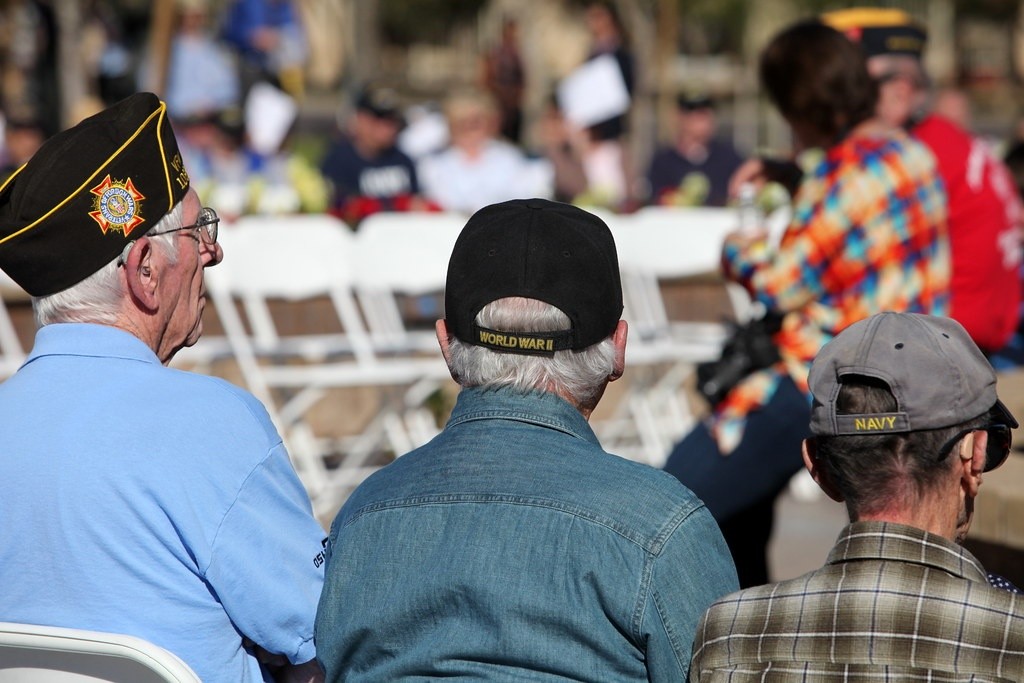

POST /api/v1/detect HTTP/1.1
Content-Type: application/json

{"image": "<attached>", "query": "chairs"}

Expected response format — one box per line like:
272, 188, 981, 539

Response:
2, 209, 726, 516
0, 622, 200, 683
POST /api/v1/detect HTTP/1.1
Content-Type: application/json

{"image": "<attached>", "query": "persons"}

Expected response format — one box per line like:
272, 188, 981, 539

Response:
868, 56, 1023, 358
0, 90, 332, 683
312, 198, 742, 683
640, 74, 744, 205
663, 16, 952, 596
687, 310, 1023, 683
1, 0, 631, 205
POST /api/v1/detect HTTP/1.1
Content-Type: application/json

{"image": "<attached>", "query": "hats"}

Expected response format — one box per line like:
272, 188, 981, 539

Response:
822, 7, 930, 56
678, 84, 713, 109
355, 78, 404, 117
1, 92, 191, 296
807, 310, 1019, 433
443, 197, 625, 352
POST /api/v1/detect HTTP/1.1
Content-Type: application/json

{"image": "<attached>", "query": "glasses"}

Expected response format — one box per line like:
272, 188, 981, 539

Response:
117, 208, 220, 268
937, 415, 1013, 472
